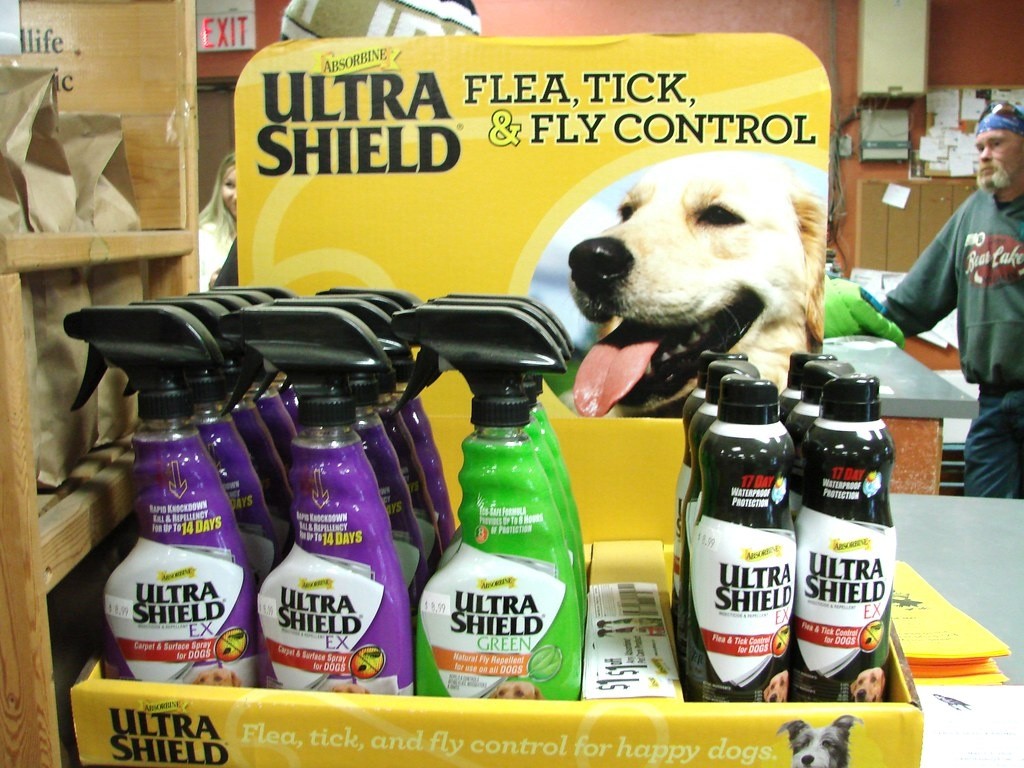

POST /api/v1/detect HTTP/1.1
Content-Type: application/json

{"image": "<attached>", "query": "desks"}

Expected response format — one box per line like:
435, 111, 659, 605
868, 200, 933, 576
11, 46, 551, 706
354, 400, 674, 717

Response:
888, 495, 1023, 766
824, 336, 979, 495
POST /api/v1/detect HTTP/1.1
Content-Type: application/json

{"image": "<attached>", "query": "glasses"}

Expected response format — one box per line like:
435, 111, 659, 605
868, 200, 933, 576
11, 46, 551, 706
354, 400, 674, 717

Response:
977, 100, 1024, 123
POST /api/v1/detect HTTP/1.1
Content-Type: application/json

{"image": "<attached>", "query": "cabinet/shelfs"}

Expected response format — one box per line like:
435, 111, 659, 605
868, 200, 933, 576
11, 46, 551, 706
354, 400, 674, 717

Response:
856, 178, 977, 268
0, 1, 204, 767
68, 22, 923, 767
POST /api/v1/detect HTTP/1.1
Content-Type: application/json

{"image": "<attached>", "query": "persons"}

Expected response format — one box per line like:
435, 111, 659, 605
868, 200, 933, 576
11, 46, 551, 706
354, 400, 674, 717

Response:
198, 150, 238, 293
879, 103, 1024, 499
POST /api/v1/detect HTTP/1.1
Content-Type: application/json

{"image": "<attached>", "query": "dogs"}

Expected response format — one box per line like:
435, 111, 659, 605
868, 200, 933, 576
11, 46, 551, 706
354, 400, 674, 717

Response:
568, 158, 829, 418
194, 669, 546, 710
763, 668, 887, 704
776, 715, 861, 768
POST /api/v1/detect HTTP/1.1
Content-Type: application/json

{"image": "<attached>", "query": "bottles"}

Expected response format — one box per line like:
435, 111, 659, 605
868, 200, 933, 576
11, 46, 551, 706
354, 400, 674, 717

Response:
675, 347, 893, 705
